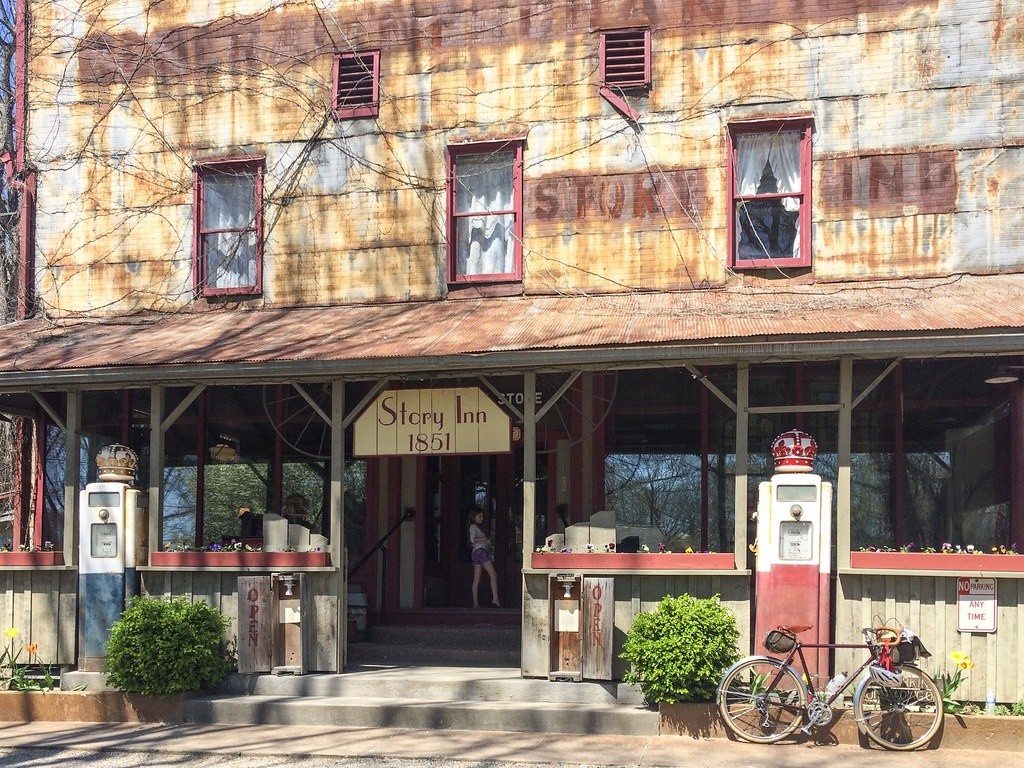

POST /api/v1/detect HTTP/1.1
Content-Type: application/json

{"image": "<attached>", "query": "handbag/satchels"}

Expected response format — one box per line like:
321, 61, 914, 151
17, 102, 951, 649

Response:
761, 627, 796, 654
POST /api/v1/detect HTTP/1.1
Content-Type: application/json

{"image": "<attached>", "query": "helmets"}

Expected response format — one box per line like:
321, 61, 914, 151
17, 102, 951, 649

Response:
869, 665, 903, 687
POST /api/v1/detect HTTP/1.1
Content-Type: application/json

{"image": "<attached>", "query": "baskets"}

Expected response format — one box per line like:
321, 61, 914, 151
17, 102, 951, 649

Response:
866, 637, 918, 664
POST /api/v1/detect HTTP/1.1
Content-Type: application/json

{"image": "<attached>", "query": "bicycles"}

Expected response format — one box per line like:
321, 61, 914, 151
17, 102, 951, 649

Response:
716, 614, 943, 750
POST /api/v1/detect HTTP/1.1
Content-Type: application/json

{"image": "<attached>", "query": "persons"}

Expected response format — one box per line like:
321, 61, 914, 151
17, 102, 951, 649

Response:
468, 507, 502, 610
